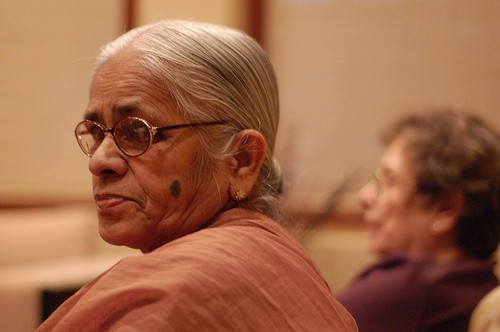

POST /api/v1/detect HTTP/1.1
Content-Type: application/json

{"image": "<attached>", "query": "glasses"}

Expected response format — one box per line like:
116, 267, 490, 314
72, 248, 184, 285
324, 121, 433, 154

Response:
73, 117, 232, 157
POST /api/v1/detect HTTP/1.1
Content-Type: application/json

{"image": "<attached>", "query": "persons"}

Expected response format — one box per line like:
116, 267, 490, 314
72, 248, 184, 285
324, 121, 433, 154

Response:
34, 18, 360, 332
333, 106, 499, 332
